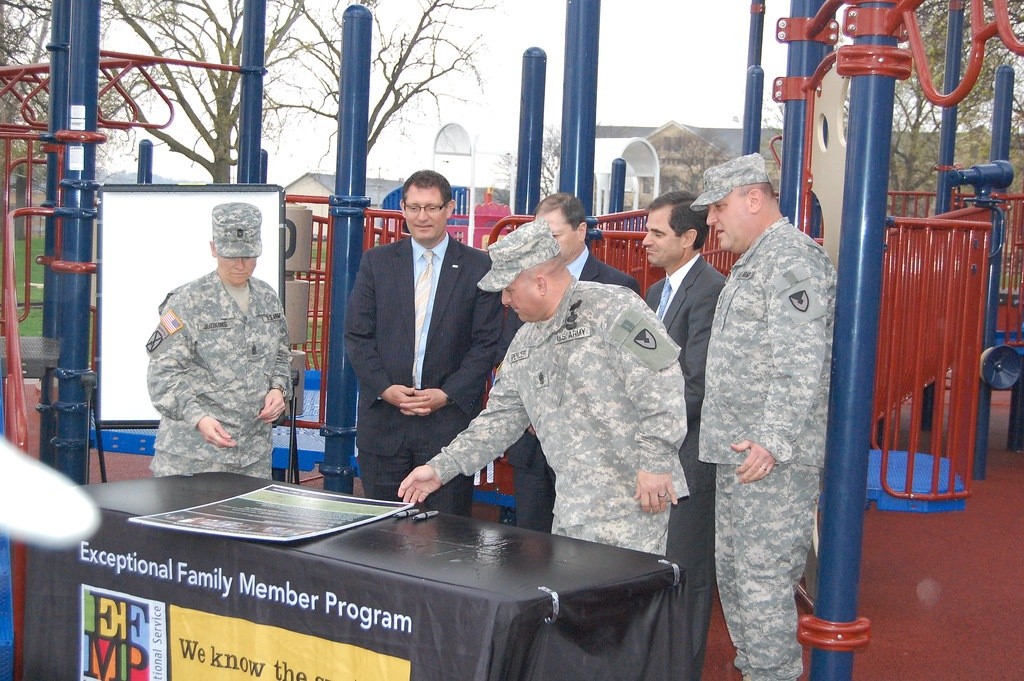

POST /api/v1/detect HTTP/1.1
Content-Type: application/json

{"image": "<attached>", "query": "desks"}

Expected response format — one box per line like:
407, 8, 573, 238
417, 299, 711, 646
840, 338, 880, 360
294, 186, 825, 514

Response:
20, 471, 686, 681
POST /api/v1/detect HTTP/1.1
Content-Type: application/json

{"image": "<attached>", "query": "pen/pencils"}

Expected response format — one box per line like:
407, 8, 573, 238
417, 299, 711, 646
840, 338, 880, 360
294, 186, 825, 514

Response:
392, 509, 419, 519
413, 511, 439, 521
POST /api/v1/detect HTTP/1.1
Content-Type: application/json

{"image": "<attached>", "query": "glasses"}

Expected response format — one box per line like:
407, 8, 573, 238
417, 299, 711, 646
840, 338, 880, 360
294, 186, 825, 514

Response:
404, 201, 449, 212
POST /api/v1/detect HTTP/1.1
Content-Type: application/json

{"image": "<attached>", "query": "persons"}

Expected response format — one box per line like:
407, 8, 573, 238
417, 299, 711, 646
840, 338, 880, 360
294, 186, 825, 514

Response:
146, 203, 294, 480
689, 152, 837, 681
495, 193, 640, 532
644, 189, 727, 681
397, 216, 690, 557
344, 170, 504, 519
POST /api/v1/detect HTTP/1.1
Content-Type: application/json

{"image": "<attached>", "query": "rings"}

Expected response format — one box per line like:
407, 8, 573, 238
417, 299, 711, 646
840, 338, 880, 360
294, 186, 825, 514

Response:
761, 465, 766, 470
276, 413, 279, 416
658, 493, 666, 497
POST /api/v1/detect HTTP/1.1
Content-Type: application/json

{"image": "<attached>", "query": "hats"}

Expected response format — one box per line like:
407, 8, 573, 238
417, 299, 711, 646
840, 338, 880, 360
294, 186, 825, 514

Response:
212, 202, 262, 258
476, 218, 561, 292
689, 152, 768, 212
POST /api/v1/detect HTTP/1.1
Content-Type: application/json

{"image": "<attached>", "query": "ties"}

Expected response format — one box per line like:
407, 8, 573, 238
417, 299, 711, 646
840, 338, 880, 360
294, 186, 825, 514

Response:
412, 251, 434, 389
658, 278, 672, 320
